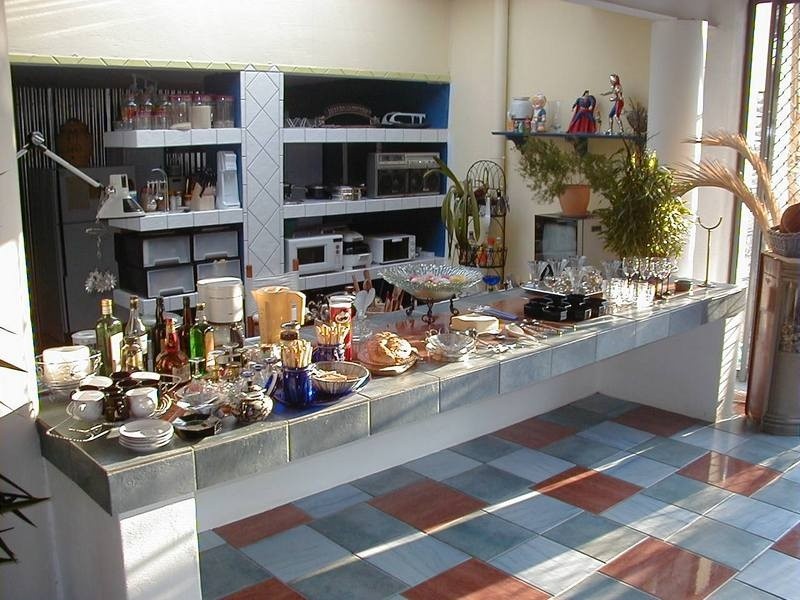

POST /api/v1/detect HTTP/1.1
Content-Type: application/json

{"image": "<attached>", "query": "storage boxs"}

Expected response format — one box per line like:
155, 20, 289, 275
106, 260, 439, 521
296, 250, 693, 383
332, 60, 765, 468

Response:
114, 227, 241, 299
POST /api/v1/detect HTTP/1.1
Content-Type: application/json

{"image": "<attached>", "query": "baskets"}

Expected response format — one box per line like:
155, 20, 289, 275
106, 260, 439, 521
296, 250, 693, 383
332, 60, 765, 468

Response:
311, 360, 369, 394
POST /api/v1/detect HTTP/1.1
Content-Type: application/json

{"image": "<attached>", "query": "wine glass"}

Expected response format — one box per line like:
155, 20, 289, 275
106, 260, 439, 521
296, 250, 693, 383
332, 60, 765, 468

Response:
600, 255, 673, 315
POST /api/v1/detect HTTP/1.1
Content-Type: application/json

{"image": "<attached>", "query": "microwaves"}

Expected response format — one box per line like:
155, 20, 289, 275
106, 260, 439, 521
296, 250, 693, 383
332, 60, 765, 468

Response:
284, 233, 343, 275
365, 234, 416, 264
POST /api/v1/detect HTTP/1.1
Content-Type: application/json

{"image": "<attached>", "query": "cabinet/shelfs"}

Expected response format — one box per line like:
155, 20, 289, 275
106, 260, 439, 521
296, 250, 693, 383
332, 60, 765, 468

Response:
455, 160, 508, 289
282, 128, 450, 316
103, 130, 244, 315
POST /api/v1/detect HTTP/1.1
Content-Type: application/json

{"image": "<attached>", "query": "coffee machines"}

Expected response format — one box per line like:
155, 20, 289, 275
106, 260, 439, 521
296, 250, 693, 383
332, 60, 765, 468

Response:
196, 277, 245, 361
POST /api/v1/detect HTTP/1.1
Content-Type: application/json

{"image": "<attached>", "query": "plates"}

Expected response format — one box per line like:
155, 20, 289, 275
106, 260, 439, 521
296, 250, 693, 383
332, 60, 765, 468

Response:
118, 414, 222, 453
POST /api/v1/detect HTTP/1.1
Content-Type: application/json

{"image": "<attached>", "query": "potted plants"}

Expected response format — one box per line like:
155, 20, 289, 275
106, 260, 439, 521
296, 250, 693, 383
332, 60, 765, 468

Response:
510, 137, 593, 216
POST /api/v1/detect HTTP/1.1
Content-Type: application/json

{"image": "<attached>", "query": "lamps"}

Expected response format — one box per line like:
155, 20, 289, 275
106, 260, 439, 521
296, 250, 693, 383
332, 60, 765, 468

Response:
16, 130, 146, 219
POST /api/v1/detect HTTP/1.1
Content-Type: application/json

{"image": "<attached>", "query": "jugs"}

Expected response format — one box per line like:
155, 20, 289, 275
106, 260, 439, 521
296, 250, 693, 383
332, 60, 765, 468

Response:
251, 286, 306, 344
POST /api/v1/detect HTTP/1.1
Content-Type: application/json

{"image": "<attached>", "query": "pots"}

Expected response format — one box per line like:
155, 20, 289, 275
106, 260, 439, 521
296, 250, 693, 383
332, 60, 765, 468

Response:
342, 231, 372, 269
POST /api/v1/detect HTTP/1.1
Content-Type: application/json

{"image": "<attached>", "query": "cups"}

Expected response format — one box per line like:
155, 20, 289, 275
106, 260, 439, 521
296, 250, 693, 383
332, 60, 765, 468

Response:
283, 364, 312, 408
315, 343, 346, 362
526, 255, 586, 294
70, 370, 168, 421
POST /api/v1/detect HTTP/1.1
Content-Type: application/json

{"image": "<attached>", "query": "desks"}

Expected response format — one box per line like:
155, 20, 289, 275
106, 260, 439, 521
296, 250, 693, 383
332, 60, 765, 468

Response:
35, 266, 748, 600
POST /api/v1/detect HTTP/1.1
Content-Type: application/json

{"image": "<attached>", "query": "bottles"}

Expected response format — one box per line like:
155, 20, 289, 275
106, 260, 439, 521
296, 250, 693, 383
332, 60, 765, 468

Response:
155, 319, 191, 390
121, 93, 235, 128
152, 296, 166, 372
96, 298, 123, 377
179, 296, 196, 359
125, 295, 149, 371
328, 296, 352, 362
121, 336, 144, 371
508, 96, 534, 119
190, 301, 214, 378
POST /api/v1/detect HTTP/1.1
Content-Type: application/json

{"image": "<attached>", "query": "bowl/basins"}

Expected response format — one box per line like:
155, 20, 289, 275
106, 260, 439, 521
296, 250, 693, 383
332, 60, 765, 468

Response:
377, 264, 483, 301
309, 361, 370, 396
426, 333, 473, 353
